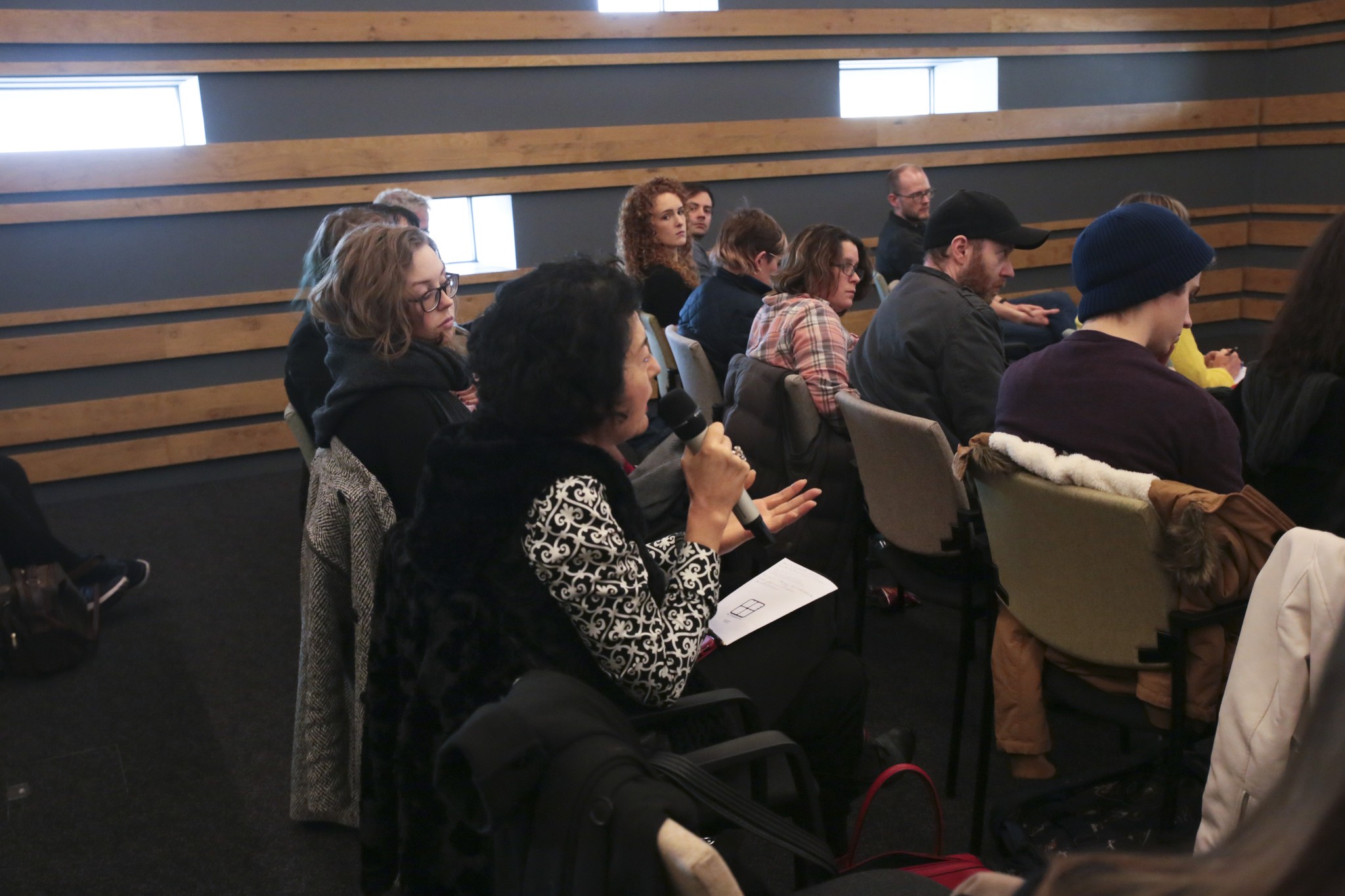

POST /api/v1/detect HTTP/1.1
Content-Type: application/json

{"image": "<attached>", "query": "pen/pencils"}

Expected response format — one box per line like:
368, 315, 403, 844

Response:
1225, 347, 1238, 356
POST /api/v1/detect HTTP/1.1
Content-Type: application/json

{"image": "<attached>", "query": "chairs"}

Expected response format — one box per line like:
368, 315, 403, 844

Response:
283, 306, 1344, 896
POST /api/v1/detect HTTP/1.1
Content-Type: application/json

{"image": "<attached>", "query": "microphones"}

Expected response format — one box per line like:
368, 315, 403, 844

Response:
656, 386, 779, 559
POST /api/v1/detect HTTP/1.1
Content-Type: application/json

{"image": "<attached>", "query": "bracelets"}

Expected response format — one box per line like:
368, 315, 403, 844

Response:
1000, 298, 1006, 303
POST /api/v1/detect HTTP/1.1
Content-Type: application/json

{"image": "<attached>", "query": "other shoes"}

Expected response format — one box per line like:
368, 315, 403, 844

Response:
850, 721, 917, 795
867, 582, 915, 608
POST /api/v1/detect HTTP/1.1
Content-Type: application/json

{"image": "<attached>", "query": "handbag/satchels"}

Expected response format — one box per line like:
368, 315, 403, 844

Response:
0, 563, 94, 679
839, 764, 994, 893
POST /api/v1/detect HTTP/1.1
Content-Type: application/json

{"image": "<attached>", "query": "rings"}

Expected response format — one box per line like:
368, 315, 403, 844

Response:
735, 450, 747, 461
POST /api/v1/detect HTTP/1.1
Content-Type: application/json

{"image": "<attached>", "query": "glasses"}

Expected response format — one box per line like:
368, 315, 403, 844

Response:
392, 273, 460, 313
756, 251, 787, 271
893, 188, 934, 203
832, 262, 865, 281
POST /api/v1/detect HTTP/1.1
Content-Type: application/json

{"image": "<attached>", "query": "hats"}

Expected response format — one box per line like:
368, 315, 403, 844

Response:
924, 189, 1050, 250
1071, 203, 1216, 322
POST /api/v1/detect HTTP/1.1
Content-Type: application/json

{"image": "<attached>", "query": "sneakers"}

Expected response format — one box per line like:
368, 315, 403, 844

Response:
90, 553, 150, 591
76, 576, 129, 613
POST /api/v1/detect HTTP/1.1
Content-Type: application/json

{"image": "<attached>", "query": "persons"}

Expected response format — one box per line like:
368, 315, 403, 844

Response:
0, 452, 150, 613
283, 164, 1345, 896
